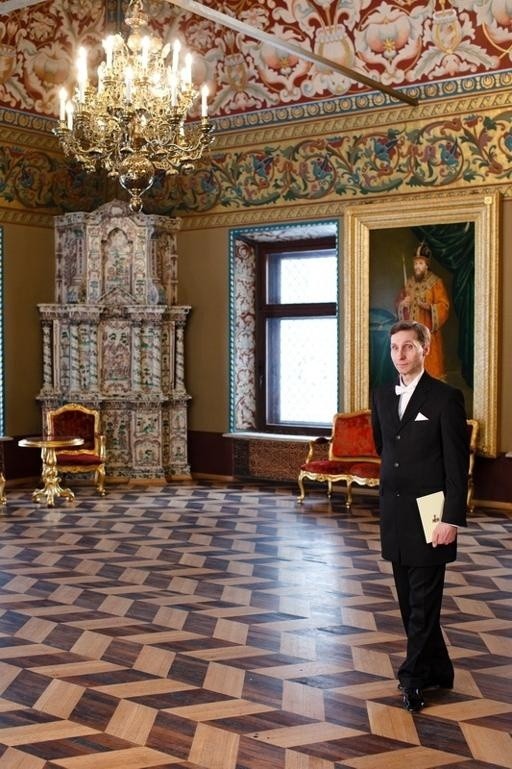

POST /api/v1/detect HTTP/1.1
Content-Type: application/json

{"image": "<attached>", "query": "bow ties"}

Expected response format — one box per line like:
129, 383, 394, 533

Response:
394, 385, 415, 397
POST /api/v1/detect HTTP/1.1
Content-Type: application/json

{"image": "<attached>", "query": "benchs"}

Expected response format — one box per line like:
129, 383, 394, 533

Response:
295, 410, 482, 519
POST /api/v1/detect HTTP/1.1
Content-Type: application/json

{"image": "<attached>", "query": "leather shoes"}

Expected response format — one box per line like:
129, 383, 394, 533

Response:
398, 677, 454, 713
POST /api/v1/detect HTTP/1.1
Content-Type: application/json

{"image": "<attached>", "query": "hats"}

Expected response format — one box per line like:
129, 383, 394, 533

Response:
412, 242, 432, 263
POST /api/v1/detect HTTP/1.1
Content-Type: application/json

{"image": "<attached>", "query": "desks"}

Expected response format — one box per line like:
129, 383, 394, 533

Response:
17, 433, 86, 509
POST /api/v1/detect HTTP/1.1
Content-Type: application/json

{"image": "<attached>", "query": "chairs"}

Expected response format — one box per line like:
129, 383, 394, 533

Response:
40, 402, 106, 497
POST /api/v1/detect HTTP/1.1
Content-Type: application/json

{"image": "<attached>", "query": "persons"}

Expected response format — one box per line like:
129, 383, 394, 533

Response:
371, 320, 468, 714
394, 255, 450, 384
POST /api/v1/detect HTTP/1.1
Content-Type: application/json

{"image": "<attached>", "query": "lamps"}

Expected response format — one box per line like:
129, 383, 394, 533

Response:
47, 1, 214, 214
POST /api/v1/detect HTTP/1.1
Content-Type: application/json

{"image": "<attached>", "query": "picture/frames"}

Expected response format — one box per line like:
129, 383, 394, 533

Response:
334, 187, 501, 461
